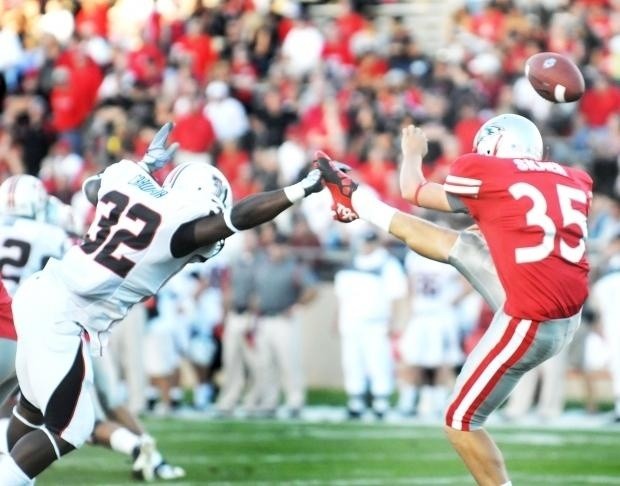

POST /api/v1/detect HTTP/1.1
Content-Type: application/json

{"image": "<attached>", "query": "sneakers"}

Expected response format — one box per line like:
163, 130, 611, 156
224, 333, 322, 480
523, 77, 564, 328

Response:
131, 433, 185, 482
312, 150, 360, 223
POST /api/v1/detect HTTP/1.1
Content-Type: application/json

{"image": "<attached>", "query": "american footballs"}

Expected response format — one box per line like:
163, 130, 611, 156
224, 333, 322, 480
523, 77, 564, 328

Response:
525, 52, 585, 103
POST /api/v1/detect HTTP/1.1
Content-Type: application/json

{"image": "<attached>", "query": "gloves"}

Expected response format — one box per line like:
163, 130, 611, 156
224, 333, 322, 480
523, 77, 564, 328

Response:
302, 161, 352, 196
141, 122, 179, 172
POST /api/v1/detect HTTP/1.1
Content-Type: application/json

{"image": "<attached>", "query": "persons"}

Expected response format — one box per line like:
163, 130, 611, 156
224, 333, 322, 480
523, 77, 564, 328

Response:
0, 172, 186, 480
313, 111, 594, 485
1, 117, 353, 484
0, 0, 620, 426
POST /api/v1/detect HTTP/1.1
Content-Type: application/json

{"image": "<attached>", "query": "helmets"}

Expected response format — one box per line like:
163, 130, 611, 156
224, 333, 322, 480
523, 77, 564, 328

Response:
472, 114, 544, 161
163, 161, 233, 213
1, 174, 49, 220
